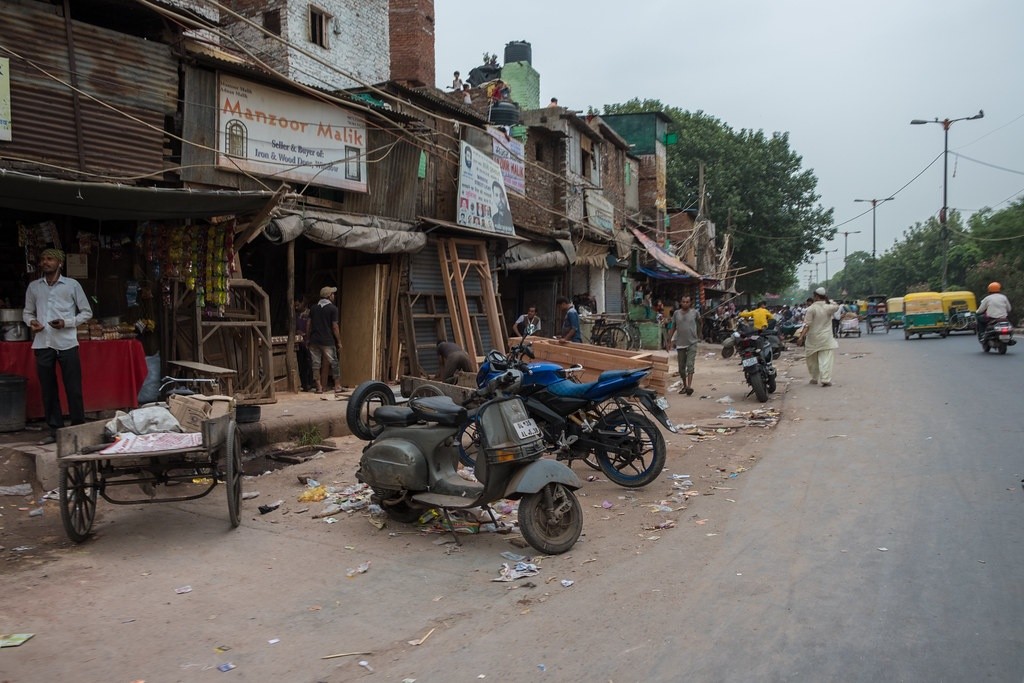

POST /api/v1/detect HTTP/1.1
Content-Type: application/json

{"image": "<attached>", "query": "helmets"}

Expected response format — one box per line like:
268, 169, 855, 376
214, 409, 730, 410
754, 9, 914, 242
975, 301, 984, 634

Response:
988, 283, 1001, 293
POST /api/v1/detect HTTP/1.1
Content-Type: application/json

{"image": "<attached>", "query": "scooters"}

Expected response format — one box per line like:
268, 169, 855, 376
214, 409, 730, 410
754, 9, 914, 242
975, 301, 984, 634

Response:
701, 305, 802, 360
976, 311, 1018, 355
355, 365, 585, 556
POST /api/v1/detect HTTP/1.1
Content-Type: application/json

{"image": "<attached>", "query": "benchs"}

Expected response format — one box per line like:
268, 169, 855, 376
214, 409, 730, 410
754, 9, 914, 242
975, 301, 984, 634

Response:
166, 359, 238, 398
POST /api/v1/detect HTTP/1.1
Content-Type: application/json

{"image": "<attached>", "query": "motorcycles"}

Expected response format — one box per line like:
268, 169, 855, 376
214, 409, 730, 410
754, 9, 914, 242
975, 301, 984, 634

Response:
901, 291, 946, 340
832, 295, 904, 335
940, 291, 978, 336
458, 323, 679, 488
732, 318, 777, 403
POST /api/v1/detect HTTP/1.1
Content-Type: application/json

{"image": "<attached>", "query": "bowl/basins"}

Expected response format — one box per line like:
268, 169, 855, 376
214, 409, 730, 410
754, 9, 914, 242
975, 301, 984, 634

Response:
235, 405, 261, 423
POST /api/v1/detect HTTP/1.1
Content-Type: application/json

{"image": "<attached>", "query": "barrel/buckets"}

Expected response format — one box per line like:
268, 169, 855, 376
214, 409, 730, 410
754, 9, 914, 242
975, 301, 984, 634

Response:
0, 374, 26, 431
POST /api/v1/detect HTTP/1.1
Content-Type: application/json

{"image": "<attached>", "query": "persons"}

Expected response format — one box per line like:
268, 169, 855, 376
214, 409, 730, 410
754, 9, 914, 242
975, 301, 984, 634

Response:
556, 296, 582, 343
705, 303, 739, 343
976, 282, 1011, 341
460, 84, 472, 106
492, 80, 505, 106
23, 249, 93, 444
766, 297, 813, 323
513, 307, 541, 337
284, 296, 312, 392
663, 296, 703, 394
739, 302, 774, 331
833, 300, 860, 338
632, 285, 653, 308
548, 98, 558, 107
654, 300, 664, 312
446, 71, 464, 91
436, 340, 471, 378
306, 287, 348, 393
460, 146, 514, 231
796, 287, 839, 386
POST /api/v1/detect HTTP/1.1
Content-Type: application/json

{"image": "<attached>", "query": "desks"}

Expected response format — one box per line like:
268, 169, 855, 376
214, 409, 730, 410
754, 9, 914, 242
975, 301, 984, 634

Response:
638, 322, 663, 349
0, 338, 149, 422
260, 342, 302, 392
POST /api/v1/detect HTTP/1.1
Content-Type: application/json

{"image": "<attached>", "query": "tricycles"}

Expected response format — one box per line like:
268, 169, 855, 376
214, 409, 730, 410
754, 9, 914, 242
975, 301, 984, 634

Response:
838, 311, 862, 338
56, 377, 246, 544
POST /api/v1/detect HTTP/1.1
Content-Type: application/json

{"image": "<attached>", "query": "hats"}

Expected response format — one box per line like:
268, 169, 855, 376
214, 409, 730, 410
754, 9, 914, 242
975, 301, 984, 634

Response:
320, 286, 337, 298
816, 287, 826, 296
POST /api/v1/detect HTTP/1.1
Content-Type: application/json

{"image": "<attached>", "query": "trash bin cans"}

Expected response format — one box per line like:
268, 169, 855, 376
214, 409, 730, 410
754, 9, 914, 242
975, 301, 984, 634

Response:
704, 316, 718, 339
0, 375, 28, 432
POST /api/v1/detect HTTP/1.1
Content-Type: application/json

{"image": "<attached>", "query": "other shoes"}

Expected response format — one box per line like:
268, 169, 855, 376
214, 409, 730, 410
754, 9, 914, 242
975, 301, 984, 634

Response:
810, 379, 818, 384
822, 382, 830, 386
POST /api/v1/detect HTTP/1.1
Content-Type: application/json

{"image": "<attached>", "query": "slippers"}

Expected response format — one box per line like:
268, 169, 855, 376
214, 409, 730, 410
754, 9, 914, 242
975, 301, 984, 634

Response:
38, 436, 55, 445
687, 388, 693, 395
335, 387, 350, 393
315, 388, 323, 393
679, 387, 686, 393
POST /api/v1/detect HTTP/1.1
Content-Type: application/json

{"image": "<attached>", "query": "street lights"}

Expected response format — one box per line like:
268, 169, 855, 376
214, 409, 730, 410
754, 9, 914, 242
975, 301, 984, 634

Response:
822, 248, 838, 281
834, 231, 862, 272
911, 114, 982, 290
810, 261, 826, 284
805, 269, 816, 284
854, 197, 895, 294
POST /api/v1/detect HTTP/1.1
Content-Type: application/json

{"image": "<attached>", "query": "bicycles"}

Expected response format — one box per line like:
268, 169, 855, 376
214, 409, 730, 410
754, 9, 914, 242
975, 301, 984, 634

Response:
949, 306, 977, 331
576, 311, 643, 352
